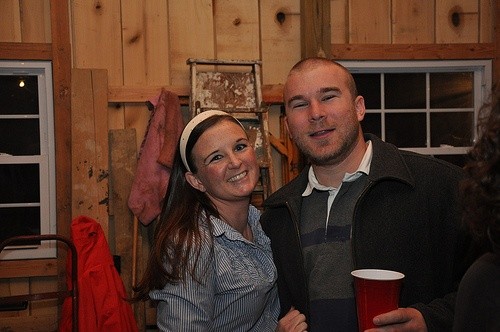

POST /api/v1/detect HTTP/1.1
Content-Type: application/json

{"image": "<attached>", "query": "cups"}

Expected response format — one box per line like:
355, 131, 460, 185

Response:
350, 269, 406, 332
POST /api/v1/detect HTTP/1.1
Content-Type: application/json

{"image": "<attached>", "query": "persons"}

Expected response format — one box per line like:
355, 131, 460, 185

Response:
452, 84, 500, 332
122, 108, 309, 332
258, 57, 465, 332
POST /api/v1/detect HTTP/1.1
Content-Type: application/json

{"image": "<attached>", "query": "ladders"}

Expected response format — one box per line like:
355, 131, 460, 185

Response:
186, 57, 276, 215
0, 235, 78, 332
